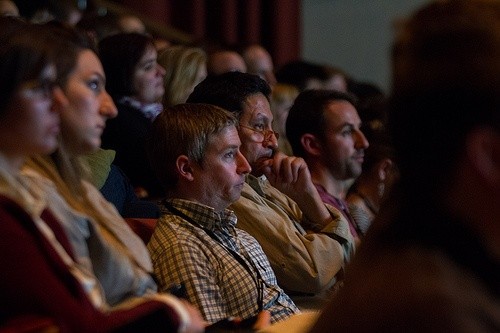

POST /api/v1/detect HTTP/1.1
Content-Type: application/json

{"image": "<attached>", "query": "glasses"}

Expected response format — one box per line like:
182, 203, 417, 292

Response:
239, 125, 279, 143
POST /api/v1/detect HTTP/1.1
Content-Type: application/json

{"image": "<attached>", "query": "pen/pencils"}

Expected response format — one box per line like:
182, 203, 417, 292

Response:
257, 279, 263, 313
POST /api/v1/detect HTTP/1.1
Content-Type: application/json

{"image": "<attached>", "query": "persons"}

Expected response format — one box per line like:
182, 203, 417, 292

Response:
18, 30, 159, 304
103, 34, 164, 165
0, 1, 500, 157
307, 1, 500, 333
185, 67, 356, 299
0, 39, 204, 333
286, 89, 371, 228
148, 104, 302, 333
